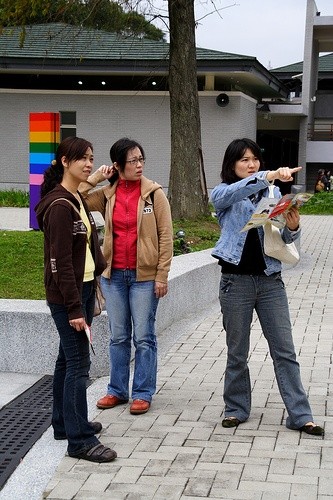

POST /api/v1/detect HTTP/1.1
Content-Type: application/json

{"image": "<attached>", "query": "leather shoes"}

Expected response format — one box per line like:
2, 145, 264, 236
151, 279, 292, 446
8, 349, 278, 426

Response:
130, 398, 149, 413
96, 394, 122, 408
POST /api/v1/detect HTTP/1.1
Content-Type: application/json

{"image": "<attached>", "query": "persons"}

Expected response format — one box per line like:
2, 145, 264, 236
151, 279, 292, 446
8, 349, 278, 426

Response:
34, 135, 117, 462
209, 138, 324, 438
77, 138, 173, 414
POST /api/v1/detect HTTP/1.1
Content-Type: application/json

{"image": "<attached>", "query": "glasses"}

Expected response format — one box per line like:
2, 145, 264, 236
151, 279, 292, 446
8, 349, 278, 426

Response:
126, 158, 144, 167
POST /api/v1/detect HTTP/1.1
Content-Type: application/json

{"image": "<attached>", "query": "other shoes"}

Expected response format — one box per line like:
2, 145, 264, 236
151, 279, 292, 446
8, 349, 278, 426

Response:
289, 425, 325, 436
222, 418, 240, 428
89, 422, 102, 434
65, 442, 117, 462
